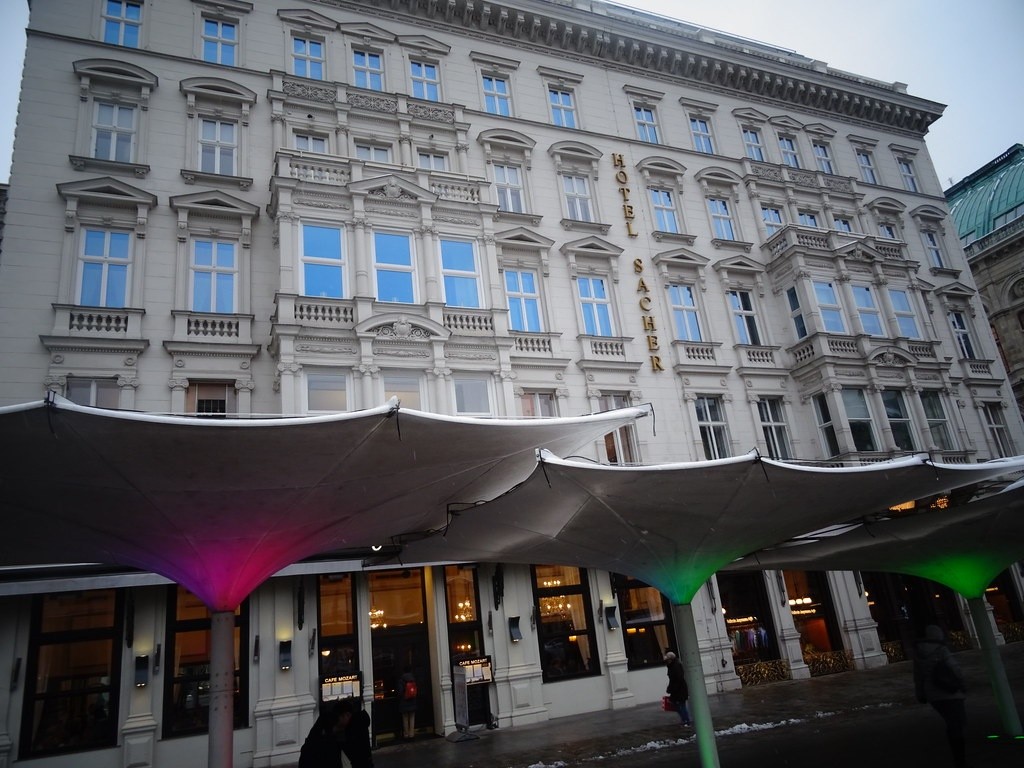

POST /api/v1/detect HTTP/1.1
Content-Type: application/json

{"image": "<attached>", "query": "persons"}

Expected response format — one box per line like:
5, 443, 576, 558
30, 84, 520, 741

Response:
912, 622, 975, 768
664, 652, 692, 728
397, 663, 417, 738
342, 697, 373, 768
299, 700, 353, 768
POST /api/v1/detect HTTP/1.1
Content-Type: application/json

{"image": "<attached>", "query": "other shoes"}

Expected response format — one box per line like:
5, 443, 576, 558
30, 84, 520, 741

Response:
408, 736, 416, 742
402, 737, 410, 743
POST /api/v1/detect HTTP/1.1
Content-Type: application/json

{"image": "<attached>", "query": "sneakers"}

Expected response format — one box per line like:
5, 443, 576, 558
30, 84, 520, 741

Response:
677, 720, 692, 728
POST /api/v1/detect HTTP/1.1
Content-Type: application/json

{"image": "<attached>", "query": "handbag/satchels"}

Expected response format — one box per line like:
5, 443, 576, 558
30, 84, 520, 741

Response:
662, 696, 674, 711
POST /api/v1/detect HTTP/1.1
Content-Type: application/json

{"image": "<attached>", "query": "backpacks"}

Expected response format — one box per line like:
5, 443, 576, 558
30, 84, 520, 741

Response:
403, 680, 417, 700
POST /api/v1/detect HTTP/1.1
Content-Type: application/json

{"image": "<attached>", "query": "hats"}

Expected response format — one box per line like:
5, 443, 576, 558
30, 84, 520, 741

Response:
663, 651, 676, 661
